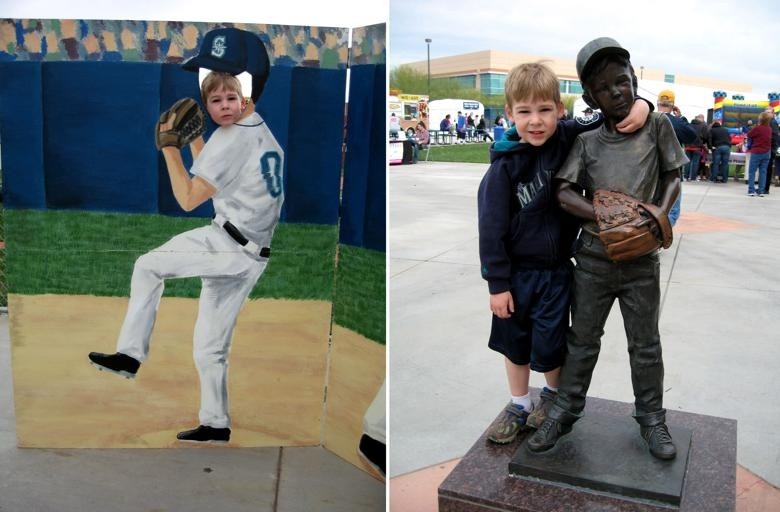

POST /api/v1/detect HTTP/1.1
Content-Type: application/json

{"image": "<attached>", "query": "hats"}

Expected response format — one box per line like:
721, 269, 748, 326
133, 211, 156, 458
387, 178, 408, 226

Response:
657, 89, 675, 103
181, 28, 270, 75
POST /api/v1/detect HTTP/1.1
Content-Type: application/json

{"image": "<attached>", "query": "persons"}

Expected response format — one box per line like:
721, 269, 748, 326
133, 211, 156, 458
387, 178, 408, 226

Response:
88, 27, 284, 443
390, 89, 780, 228
524, 37, 692, 460
357, 377, 386, 475
476, 63, 654, 445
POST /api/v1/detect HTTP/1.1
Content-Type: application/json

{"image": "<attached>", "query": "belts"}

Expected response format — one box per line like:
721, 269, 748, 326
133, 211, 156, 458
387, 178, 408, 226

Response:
212, 213, 270, 257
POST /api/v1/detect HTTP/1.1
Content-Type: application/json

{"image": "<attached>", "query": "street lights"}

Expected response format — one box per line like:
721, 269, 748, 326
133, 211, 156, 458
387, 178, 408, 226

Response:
639, 64, 646, 82
424, 36, 433, 97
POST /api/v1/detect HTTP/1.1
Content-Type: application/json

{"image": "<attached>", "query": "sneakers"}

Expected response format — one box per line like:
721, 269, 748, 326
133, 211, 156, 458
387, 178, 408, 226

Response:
175, 427, 232, 445
680, 175, 731, 181
746, 191, 770, 196
487, 399, 536, 444
87, 350, 141, 378
357, 433, 387, 478
527, 386, 556, 429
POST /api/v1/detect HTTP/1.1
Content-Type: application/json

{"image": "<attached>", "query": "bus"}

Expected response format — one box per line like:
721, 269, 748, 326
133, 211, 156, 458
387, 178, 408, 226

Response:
389, 89, 430, 138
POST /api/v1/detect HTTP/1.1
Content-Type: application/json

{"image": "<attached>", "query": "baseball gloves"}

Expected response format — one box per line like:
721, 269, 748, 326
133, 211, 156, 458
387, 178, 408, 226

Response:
155, 97, 204, 148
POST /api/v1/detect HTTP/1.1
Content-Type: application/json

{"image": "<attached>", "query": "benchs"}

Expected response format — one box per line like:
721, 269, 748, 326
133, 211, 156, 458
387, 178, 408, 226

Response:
424, 125, 489, 145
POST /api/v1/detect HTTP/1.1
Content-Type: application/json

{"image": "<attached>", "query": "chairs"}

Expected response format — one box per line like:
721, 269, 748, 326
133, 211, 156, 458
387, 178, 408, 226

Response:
414, 138, 432, 163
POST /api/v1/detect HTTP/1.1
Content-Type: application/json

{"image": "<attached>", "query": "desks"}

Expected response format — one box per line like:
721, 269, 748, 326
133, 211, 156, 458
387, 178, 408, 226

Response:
389, 139, 413, 167
704, 151, 748, 182
